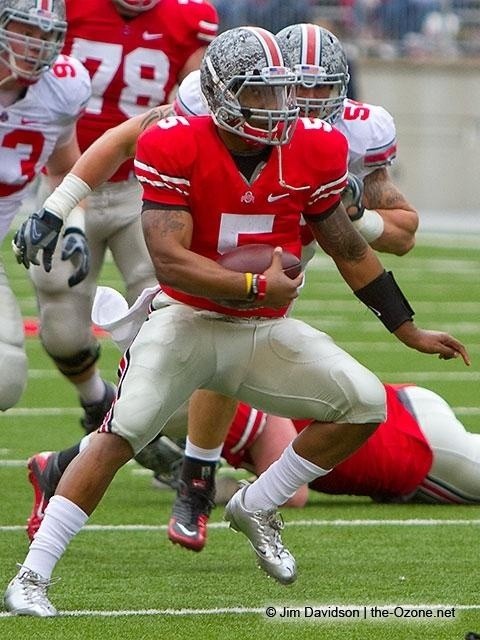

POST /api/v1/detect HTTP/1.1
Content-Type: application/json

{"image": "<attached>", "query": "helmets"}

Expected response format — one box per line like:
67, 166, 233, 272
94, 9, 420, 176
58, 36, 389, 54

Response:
197, 23, 298, 144
1, 1, 71, 84
275, 21, 352, 117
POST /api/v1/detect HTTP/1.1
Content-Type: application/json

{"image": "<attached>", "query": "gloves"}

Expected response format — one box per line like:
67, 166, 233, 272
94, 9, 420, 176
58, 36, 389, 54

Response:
59, 232, 91, 287
345, 173, 366, 220
11, 210, 58, 272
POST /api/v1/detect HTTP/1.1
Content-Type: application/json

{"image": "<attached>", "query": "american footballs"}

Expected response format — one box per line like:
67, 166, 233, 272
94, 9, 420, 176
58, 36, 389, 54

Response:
216, 244, 302, 280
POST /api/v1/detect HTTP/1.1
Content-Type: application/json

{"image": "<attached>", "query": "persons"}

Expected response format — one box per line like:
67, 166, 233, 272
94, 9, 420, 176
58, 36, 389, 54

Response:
30, 0, 221, 475
13, 20, 420, 552
213, 382, 480, 524
0, 0, 93, 414
4, 27, 472, 618
218, 0, 480, 64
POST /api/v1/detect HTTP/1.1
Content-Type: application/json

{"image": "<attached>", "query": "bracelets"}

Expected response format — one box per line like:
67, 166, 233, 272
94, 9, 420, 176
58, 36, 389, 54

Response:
354, 269, 415, 333
245, 272, 267, 303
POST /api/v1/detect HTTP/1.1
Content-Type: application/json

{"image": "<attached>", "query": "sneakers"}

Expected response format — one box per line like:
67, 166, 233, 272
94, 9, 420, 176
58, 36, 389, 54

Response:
84, 380, 192, 489
4, 575, 56, 619
27, 450, 58, 542
224, 482, 298, 586
167, 472, 215, 551
